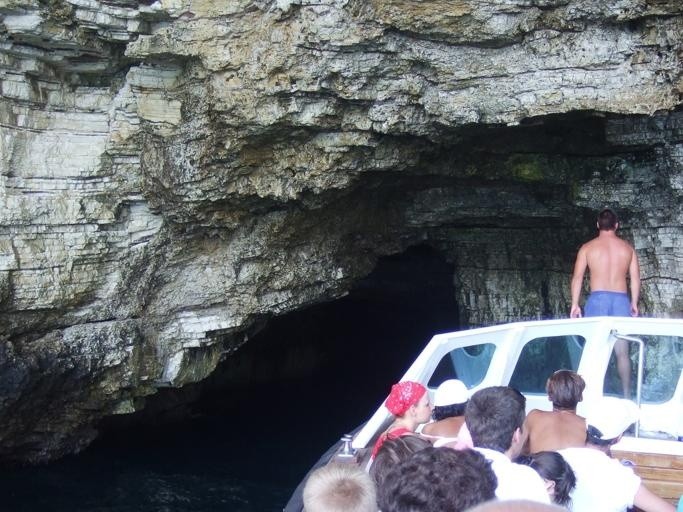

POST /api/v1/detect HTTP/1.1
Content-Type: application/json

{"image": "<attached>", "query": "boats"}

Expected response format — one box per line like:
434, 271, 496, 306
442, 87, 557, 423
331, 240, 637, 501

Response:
280, 315, 683, 510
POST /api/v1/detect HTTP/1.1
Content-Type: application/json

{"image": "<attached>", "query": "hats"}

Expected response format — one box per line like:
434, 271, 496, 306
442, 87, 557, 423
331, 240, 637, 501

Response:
386, 381, 426, 415
434, 379, 468, 406
586, 396, 641, 440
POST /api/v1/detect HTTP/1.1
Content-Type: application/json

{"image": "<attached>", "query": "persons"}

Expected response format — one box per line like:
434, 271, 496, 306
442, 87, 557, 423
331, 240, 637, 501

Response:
521, 450, 575, 508
369, 436, 439, 486
368, 379, 435, 466
415, 378, 476, 449
521, 368, 593, 455
463, 386, 552, 504
567, 208, 641, 404
301, 465, 384, 512
554, 399, 680, 512
378, 447, 498, 512
